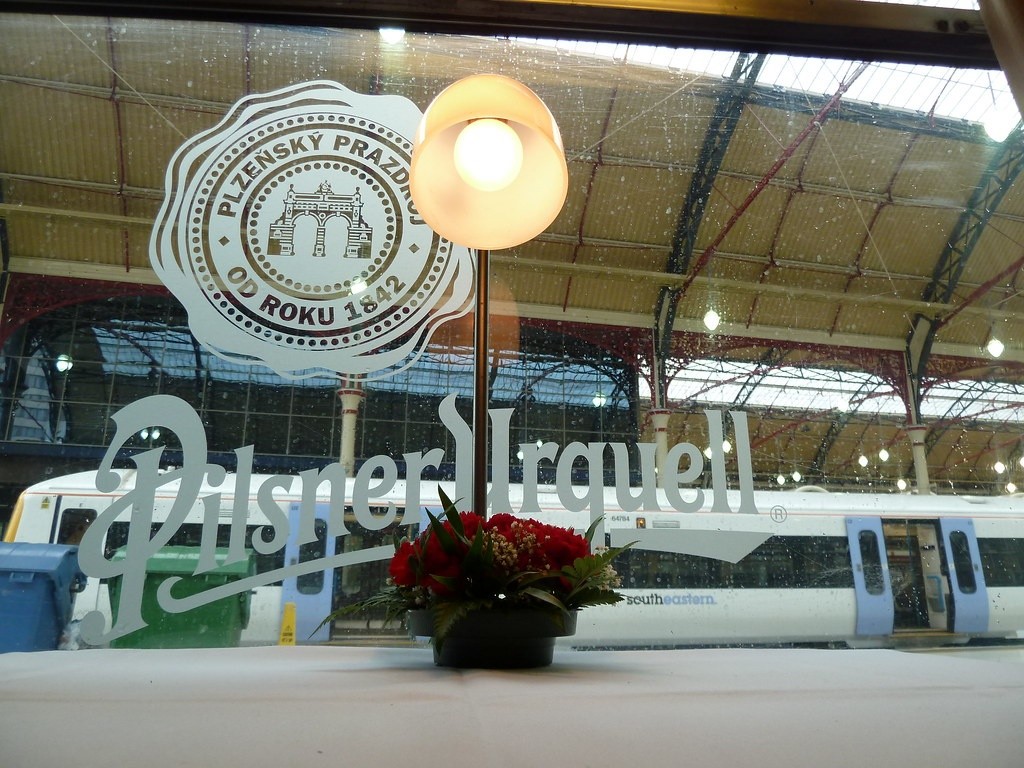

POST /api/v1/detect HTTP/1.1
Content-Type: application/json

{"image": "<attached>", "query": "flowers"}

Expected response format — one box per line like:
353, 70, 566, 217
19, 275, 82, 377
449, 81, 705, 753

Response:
307, 483, 643, 641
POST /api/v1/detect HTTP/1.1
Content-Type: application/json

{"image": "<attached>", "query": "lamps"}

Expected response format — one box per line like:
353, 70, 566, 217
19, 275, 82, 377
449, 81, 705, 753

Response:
408, 72, 573, 519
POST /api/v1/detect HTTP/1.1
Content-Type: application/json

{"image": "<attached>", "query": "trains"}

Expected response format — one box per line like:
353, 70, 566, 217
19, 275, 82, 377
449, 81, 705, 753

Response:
4, 469, 1023, 643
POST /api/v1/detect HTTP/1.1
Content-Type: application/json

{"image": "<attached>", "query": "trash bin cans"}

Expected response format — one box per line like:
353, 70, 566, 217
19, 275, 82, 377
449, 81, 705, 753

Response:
107, 544, 257, 649
0, 541, 89, 654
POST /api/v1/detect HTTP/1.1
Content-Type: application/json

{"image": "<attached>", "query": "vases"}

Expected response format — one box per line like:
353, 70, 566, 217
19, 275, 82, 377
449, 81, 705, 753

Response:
406, 608, 581, 671
